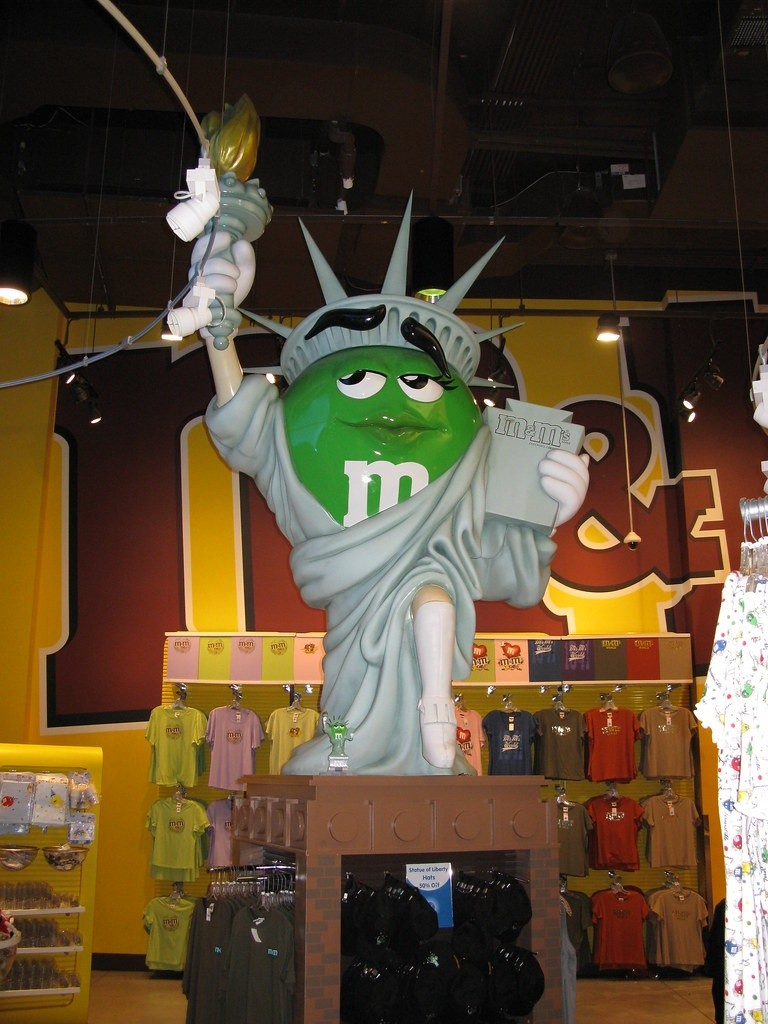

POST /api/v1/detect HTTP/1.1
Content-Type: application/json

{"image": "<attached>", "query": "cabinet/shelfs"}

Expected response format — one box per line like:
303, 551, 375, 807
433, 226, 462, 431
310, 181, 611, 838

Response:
0, 742, 102, 1024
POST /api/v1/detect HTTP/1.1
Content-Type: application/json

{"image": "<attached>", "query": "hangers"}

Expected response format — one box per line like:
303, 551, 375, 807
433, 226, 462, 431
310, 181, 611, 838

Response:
737, 495, 767, 585
164, 685, 693, 915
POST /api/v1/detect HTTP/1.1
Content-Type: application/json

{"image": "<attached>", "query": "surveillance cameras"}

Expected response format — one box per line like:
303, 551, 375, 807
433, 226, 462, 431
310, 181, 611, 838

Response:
623, 531, 641, 551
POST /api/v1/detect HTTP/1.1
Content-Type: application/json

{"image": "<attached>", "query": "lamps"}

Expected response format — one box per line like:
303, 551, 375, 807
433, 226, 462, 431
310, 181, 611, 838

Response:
704, 358, 724, 392
482, 357, 508, 406
160, 313, 183, 341
0, 218, 39, 307
682, 377, 702, 410
674, 400, 696, 423
594, 314, 621, 343
405, 212, 456, 302
55, 352, 102, 424
